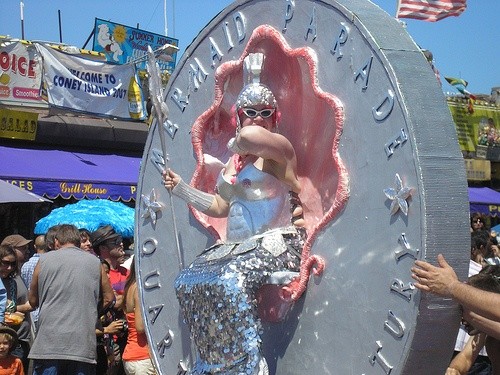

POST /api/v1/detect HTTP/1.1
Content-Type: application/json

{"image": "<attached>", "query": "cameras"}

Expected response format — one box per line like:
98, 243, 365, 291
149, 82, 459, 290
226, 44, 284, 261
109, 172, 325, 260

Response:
117, 319, 128, 332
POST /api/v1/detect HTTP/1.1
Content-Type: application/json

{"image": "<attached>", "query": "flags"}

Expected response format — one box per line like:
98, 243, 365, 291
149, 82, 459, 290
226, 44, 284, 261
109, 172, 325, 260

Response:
398, 0, 467, 23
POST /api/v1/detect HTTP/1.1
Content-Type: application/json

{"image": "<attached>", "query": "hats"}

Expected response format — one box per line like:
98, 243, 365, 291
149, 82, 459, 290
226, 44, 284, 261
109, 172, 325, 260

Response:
1, 235, 33, 248
0, 322, 18, 352
235, 52, 277, 107
90, 225, 122, 247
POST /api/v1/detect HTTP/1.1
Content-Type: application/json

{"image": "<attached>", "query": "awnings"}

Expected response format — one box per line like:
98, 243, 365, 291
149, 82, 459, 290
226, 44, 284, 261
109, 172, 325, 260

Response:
0, 140, 144, 203
468, 186, 500, 216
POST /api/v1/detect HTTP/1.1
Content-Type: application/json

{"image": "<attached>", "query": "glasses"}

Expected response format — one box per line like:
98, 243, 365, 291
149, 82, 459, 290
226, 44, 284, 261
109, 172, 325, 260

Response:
241, 107, 276, 119
105, 237, 123, 245
473, 221, 482, 223
2, 260, 16, 267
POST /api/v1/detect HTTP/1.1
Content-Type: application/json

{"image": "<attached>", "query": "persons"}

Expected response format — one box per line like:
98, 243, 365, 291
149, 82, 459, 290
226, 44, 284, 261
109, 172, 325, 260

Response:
122, 255, 155, 375
30, 224, 115, 375
92, 225, 131, 375
410, 254, 500, 341
445, 265, 500, 375
1, 235, 36, 375
0, 325, 25, 375
453, 212, 500, 375
0, 245, 18, 327
21, 235, 47, 328
160, 82, 301, 242
78, 228, 93, 252
